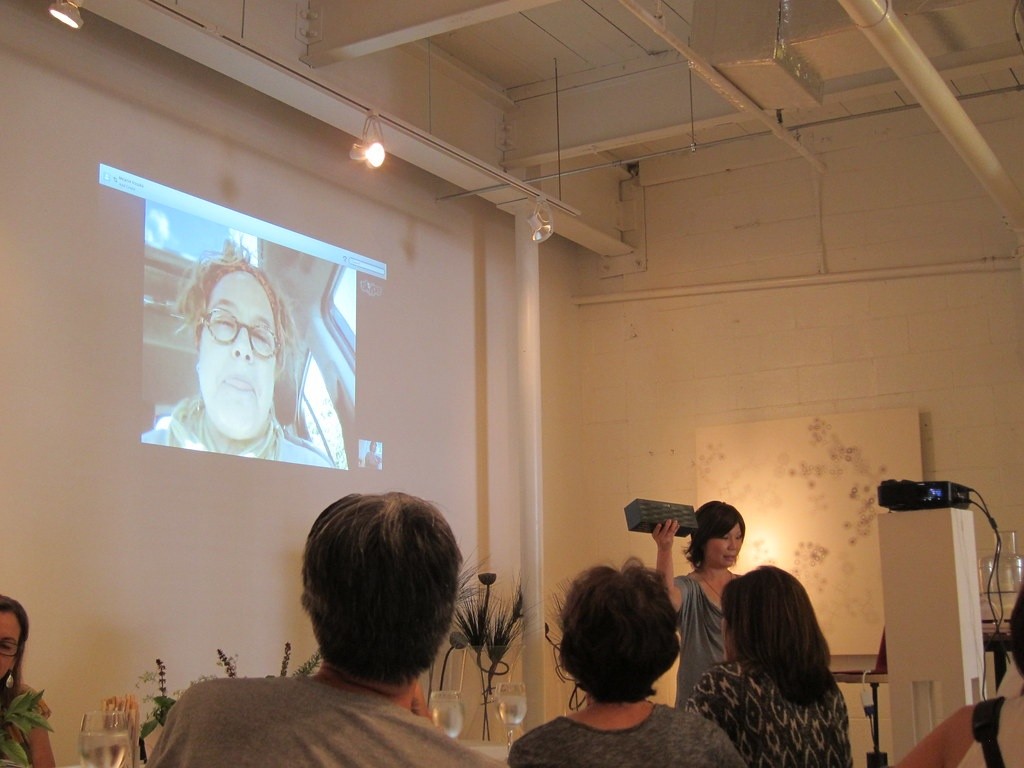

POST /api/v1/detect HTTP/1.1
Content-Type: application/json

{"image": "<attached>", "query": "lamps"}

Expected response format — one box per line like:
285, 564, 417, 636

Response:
47, 0, 84, 30
525, 197, 555, 243
348, 115, 386, 169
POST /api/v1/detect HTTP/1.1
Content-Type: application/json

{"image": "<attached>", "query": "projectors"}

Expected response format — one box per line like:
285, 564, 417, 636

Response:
877, 479, 970, 509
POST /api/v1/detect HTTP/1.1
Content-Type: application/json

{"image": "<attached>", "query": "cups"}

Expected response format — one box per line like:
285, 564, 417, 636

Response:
79, 711, 131, 767
430, 690, 465, 738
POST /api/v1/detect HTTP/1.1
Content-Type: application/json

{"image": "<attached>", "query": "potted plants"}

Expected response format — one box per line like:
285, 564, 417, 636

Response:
452, 558, 546, 761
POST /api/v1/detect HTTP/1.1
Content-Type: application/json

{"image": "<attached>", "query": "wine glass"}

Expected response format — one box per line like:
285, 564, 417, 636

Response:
493, 681, 527, 757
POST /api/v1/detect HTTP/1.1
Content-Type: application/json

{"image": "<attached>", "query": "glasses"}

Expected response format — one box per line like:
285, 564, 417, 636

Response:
198, 310, 280, 357
0, 640, 19, 656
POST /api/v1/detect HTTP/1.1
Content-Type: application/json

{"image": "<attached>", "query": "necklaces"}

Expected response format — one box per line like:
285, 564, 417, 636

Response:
697, 570, 733, 599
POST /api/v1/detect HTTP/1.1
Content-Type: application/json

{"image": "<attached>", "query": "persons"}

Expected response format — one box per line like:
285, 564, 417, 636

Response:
0, 594, 55, 768
140, 237, 335, 467
505, 555, 751, 768
653, 500, 749, 711
364, 441, 382, 469
687, 564, 1024, 768
144, 491, 511, 768
